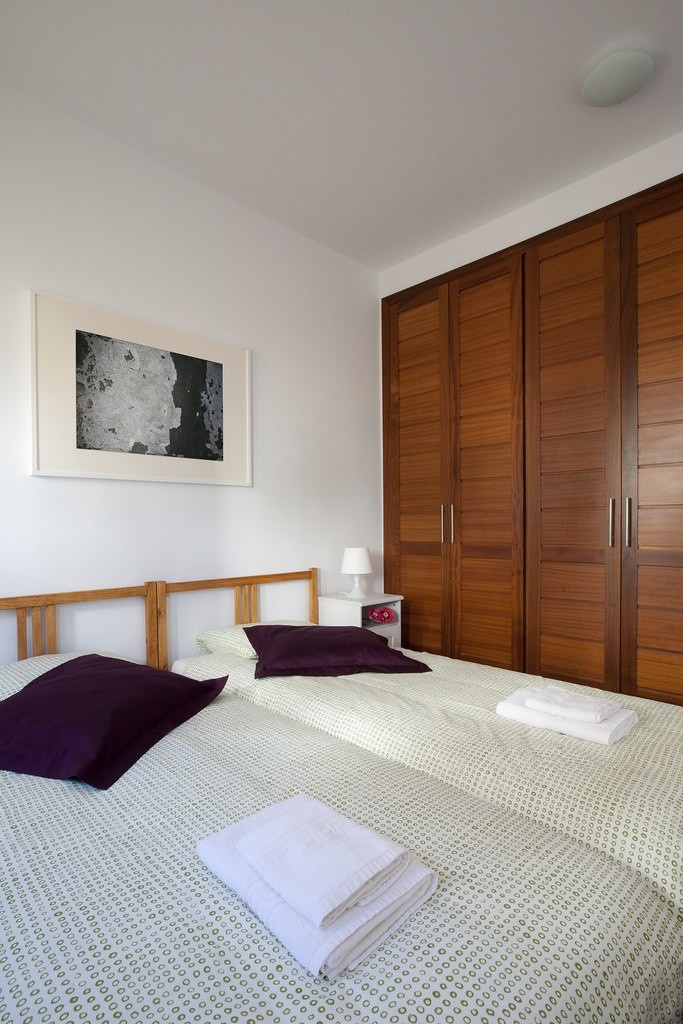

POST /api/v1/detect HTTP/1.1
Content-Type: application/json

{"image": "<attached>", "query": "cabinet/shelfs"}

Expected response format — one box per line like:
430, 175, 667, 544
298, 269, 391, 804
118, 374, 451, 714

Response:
381, 174, 682, 708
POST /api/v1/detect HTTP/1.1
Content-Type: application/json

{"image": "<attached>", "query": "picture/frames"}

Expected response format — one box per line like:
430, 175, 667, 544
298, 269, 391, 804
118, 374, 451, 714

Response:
26, 287, 253, 487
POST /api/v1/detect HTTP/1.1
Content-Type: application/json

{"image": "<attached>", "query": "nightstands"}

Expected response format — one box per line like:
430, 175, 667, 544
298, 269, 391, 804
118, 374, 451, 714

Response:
318, 594, 404, 647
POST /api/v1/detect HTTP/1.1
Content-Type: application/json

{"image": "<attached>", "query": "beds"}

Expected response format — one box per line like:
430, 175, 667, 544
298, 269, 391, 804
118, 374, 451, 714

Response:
0, 646, 683, 1024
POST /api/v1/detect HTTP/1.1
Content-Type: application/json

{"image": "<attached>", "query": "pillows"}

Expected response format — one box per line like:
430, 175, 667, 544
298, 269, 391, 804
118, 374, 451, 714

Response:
0, 620, 434, 791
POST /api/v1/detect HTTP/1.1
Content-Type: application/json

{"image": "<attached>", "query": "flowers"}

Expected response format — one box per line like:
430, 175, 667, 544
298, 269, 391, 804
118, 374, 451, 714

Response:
366, 607, 395, 624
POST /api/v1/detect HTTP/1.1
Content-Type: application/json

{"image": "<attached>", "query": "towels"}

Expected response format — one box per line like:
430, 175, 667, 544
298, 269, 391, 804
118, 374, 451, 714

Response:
195, 793, 439, 980
497, 685, 640, 747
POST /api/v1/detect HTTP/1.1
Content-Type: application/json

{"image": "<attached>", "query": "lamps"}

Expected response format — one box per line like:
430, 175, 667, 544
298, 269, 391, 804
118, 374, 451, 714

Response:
340, 547, 373, 599
